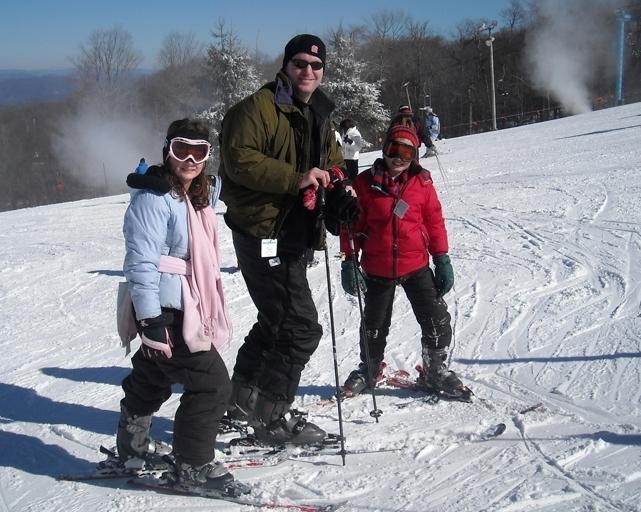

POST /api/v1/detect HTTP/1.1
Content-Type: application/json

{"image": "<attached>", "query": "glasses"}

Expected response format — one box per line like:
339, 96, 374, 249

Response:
163, 136, 211, 164
288, 56, 324, 71
381, 141, 418, 162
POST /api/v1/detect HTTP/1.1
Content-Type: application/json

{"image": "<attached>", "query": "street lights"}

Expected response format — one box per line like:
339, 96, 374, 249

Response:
608, 5, 634, 108
475, 19, 501, 131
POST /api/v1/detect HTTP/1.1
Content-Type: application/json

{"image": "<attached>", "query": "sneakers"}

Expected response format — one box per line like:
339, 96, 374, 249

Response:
259, 419, 327, 443
229, 406, 296, 425
175, 460, 253, 497
345, 364, 381, 392
421, 371, 467, 397
119, 442, 177, 465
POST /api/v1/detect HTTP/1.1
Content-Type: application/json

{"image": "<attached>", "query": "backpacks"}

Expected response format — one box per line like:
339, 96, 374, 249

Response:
401, 116, 416, 130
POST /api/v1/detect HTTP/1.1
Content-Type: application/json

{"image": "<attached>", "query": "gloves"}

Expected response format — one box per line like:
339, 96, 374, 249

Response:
433, 254, 455, 297
138, 317, 176, 360
340, 256, 368, 298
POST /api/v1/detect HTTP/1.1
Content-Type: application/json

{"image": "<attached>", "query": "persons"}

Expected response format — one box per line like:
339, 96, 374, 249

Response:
330, 120, 343, 149
135, 158, 149, 174
119, 117, 233, 486
339, 119, 373, 178
386, 105, 436, 152
340, 124, 464, 398
419, 106, 441, 158
217, 34, 357, 445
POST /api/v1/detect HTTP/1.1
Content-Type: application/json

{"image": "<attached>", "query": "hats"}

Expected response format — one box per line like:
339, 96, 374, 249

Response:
163, 118, 211, 167
282, 33, 326, 77
398, 104, 433, 113
386, 125, 420, 150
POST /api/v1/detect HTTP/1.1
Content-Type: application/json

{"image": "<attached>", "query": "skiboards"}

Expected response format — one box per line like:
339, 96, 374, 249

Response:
313, 362, 543, 416
217, 389, 507, 459
58, 450, 348, 511
